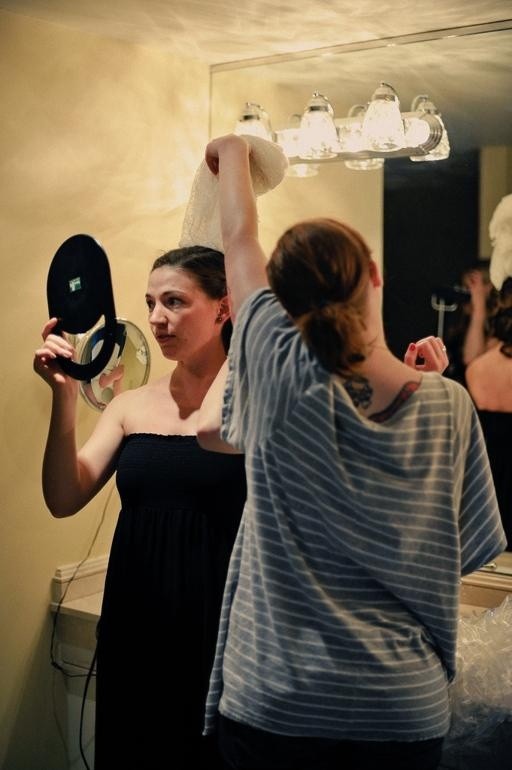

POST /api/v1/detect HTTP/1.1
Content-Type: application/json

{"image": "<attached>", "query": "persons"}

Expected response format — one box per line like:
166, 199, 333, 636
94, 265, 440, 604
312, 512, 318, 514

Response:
33, 246, 247, 770
201, 135, 507, 770
464, 271, 511, 553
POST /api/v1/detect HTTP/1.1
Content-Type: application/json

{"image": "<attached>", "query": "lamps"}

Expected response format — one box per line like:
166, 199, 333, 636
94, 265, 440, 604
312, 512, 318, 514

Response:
236, 81, 451, 178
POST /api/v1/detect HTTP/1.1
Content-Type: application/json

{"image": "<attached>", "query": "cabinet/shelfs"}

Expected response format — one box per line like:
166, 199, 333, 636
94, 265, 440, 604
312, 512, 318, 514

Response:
51, 590, 511, 769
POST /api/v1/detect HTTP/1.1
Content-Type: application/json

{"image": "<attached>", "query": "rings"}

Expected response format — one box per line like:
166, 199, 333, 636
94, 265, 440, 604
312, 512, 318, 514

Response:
441, 345, 448, 354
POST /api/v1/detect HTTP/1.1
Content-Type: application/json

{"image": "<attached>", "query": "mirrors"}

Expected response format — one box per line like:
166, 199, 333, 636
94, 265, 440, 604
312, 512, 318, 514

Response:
44, 237, 116, 382
210, 16, 512, 592
